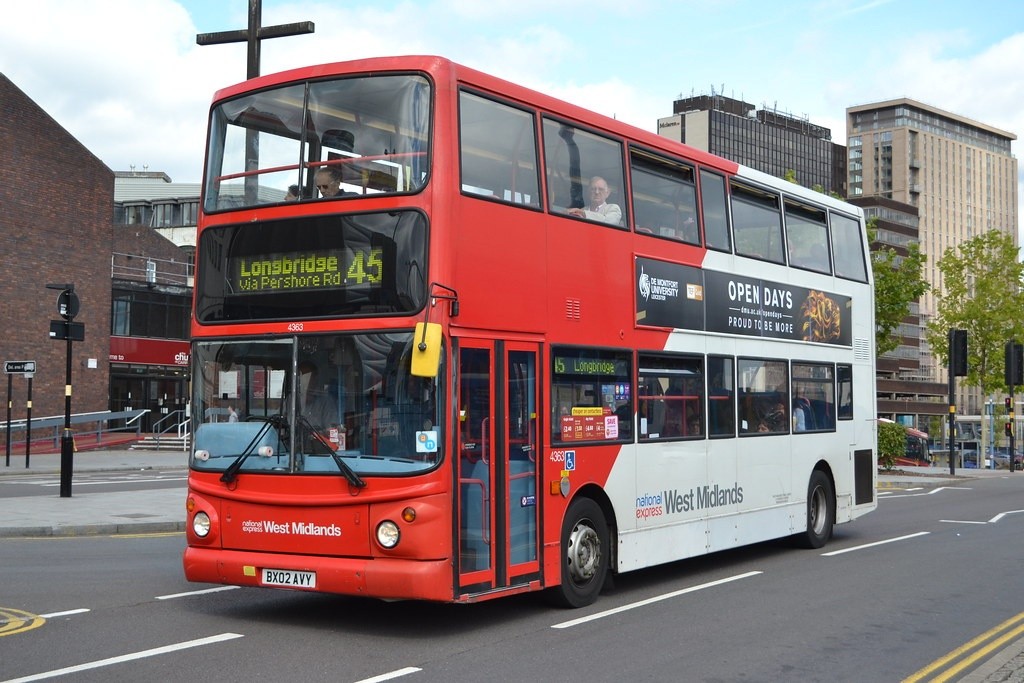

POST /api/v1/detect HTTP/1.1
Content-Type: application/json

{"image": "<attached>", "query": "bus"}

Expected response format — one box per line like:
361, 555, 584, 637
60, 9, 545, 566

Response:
183, 55, 877, 609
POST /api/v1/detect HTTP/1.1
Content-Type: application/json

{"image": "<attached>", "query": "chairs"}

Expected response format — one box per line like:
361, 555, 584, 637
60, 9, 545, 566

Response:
751, 395, 774, 425
794, 396, 817, 430
777, 400, 789, 431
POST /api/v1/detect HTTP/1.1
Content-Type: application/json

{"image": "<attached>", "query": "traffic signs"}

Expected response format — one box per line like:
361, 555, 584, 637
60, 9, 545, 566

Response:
3, 361, 36, 374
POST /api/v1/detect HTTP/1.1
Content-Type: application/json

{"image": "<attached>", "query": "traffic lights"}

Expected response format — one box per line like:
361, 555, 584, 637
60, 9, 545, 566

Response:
1005, 397, 1013, 409
1005, 422, 1013, 436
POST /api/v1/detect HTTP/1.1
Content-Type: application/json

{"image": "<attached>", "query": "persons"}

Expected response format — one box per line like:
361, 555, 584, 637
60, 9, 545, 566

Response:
273, 361, 341, 453
228, 405, 239, 422
376, 352, 521, 460
807, 243, 831, 271
284, 167, 359, 202
559, 406, 570, 416
613, 385, 788, 440
568, 176, 622, 226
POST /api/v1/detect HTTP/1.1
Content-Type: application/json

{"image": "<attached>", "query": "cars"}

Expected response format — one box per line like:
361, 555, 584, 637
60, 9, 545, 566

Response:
964, 450, 1009, 467
994, 452, 1011, 461
1013, 454, 1023, 461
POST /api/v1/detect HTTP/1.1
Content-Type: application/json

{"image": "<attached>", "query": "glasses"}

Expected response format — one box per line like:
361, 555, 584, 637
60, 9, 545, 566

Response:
315, 181, 335, 190
